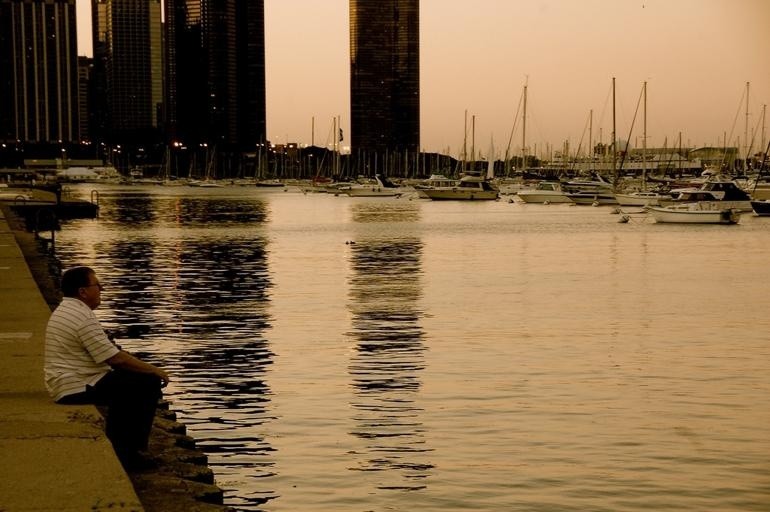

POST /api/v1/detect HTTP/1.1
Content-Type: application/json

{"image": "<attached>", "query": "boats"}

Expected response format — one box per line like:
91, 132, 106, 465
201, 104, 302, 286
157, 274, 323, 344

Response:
0, 164, 130, 193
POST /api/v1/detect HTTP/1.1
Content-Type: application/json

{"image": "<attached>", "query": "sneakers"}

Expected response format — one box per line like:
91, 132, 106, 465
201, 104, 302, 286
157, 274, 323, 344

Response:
117, 447, 167, 474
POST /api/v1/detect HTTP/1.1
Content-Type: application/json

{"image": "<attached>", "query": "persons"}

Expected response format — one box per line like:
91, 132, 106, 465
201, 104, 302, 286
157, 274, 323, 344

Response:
43, 267, 169, 474
